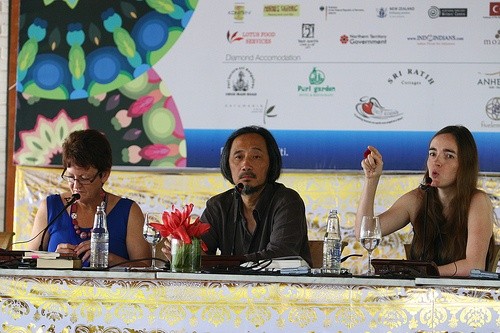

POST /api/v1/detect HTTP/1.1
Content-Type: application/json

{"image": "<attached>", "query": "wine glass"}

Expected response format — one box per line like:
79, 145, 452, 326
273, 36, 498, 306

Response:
360, 216, 382, 276
143, 212, 165, 270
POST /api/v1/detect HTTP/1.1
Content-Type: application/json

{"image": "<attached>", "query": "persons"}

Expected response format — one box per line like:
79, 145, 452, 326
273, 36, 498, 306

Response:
161, 125, 314, 276
26, 129, 153, 271
355, 125, 496, 277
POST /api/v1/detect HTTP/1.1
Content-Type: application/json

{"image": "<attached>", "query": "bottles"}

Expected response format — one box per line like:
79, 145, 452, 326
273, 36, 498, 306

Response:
322, 210, 342, 275
90, 206, 109, 268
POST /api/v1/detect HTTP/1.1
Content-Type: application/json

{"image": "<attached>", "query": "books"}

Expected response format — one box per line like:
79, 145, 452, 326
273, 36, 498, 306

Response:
109, 265, 159, 272
258, 256, 311, 269
22, 251, 82, 269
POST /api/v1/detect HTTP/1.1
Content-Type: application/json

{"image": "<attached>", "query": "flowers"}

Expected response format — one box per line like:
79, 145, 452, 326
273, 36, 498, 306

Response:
147, 204, 211, 268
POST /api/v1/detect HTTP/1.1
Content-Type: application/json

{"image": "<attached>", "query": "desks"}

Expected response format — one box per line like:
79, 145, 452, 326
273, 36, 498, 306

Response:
0, 269, 500, 333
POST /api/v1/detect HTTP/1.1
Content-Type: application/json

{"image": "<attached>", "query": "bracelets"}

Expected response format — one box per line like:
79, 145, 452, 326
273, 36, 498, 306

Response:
451, 261, 457, 277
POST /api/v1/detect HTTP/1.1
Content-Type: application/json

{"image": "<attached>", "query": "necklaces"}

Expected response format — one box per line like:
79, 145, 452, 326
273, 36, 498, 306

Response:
70, 187, 109, 238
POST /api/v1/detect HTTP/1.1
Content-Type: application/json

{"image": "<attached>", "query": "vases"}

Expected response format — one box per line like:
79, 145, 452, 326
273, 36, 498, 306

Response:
171, 238, 201, 273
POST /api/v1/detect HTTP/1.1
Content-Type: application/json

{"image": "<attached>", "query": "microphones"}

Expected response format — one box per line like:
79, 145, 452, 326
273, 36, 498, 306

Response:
419, 183, 430, 261
39, 193, 80, 250
231, 183, 244, 256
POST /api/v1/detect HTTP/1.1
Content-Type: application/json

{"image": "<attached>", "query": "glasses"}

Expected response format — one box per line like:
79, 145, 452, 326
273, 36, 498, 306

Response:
61, 168, 100, 185
